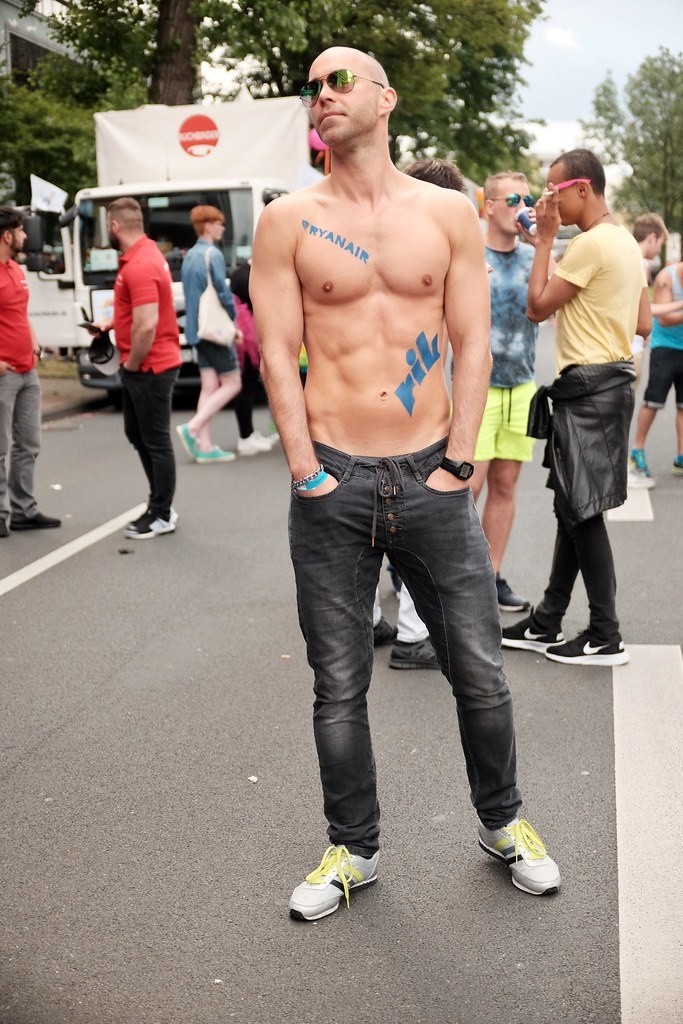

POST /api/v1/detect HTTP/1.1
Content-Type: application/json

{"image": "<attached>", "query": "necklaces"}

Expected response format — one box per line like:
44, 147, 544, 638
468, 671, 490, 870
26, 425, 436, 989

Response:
586, 213, 611, 234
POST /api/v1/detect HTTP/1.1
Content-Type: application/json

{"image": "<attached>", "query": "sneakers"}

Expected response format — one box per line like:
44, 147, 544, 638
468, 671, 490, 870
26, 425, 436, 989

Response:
477, 815, 561, 895
196, 444, 236, 463
628, 449, 650, 476
0, 517, 9, 537
496, 579, 529, 611
502, 613, 566, 655
125, 506, 179, 539
176, 424, 195, 459
389, 640, 441, 669
546, 629, 630, 666
672, 456, 683, 474
290, 844, 379, 921
9, 513, 61, 530
373, 616, 399, 647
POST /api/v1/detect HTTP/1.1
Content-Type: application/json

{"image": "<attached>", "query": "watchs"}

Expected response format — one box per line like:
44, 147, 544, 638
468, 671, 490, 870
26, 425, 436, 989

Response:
440, 457, 475, 481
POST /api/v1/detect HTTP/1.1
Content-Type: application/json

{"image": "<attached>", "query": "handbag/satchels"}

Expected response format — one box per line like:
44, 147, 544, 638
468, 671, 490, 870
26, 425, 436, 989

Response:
197, 246, 236, 346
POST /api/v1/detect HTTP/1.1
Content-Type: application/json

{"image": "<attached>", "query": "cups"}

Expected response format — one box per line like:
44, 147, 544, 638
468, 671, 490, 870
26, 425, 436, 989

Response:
515, 207, 537, 236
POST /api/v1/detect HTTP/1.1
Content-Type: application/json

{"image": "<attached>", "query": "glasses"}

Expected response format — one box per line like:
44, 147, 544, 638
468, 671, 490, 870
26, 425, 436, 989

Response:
490, 193, 536, 207
300, 69, 385, 108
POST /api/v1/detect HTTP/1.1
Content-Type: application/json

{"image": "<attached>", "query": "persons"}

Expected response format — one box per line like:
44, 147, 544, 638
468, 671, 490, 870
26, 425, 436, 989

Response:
246, 48, 560, 922
0, 149, 683, 670
87, 198, 182, 540
0, 207, 61, 539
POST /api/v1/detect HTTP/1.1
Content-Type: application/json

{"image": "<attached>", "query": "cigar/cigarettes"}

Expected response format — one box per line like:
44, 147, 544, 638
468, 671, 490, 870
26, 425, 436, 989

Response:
543, 192, 554, 196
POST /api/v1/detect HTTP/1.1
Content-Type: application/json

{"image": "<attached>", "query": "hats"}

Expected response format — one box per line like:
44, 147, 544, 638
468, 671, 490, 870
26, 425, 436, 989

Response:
89, 337, 121, 376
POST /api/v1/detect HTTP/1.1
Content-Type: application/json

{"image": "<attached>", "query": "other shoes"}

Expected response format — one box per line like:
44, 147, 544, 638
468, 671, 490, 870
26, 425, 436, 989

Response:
627, 470, 656, 488
237, 431, 280, 456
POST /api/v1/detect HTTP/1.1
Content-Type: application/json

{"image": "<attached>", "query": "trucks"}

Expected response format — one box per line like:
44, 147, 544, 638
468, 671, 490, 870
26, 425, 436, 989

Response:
11, 181, 295, 409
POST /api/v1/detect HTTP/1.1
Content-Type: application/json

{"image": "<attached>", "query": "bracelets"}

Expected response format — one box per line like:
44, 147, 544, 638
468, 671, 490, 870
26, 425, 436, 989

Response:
292, 464, 324, 488
295, 472, 328, 491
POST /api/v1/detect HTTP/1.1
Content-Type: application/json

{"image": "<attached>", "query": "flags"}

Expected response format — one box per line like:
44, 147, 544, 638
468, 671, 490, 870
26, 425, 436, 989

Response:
31, 175, 69, 213
